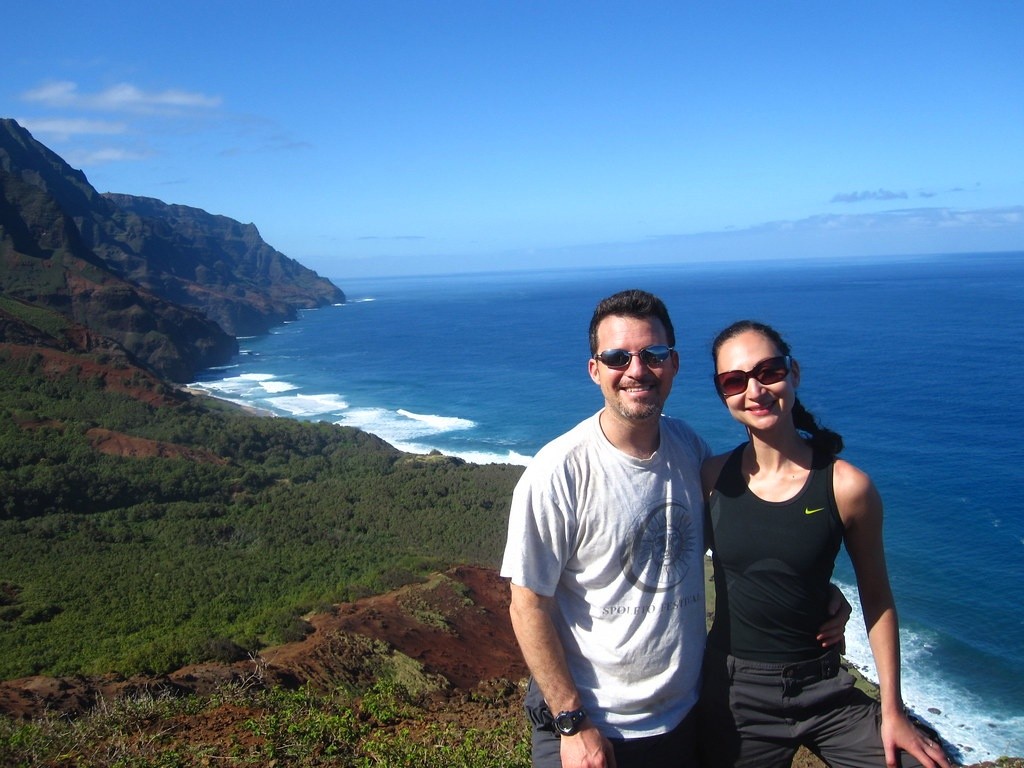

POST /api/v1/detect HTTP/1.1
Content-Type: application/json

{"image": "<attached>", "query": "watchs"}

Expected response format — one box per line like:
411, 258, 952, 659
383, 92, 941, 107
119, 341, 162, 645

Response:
550, 705, 587, 735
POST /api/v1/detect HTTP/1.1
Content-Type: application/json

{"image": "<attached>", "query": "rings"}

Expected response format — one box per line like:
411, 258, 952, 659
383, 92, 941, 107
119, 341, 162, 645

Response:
927, 738, 933, 747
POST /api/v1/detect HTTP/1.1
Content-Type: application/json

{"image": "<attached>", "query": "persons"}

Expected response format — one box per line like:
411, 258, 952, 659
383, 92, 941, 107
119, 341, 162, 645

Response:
697, 320, 954, 768
496, 288, 855, 768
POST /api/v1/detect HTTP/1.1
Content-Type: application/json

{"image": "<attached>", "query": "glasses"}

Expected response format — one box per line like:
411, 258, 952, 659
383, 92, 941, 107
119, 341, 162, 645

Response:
714, 356, 792, 397
594, 345, 674, 369
